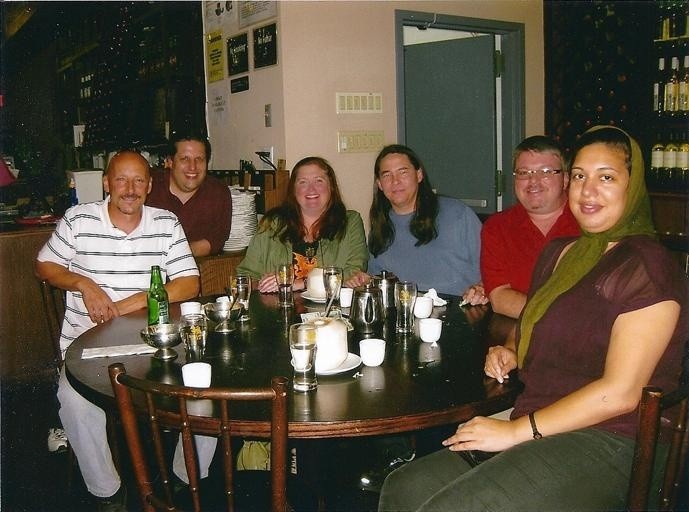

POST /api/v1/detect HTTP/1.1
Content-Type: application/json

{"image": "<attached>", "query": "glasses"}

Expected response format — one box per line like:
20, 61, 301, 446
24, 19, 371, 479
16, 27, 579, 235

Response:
509, 167, 562, 179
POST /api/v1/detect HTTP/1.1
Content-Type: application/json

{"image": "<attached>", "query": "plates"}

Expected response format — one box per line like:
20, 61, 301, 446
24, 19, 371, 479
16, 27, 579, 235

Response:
221, 189, 260, 254
300, 300, 325, 311
214, 296, 232, 303
287, 354, 362, 376
301, 310, 355, 332
299, 291, 327, 305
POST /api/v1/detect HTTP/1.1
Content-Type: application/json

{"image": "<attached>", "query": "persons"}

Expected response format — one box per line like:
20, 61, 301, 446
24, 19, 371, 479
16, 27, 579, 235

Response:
141, 135, 232, 258
345, 145, 489, 307
480, 135, 582, 318
33, 150, 219, 511
235, 156, 366, 294
379, 126, 687, 510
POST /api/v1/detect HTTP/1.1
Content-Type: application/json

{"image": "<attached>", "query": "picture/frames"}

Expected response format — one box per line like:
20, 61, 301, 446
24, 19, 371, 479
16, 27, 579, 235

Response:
226, 30, 250, 76
237, 1, 278, 29
252, 20, 279, 71
228, 73, 251, 96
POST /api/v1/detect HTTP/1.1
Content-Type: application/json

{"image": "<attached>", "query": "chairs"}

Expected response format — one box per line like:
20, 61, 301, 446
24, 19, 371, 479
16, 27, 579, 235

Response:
107, 363, 326, 512
40, 279, 165, 484
625, 385, 689, 512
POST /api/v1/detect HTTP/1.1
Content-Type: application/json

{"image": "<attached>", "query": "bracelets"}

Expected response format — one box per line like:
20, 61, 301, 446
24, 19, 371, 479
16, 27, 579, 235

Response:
302, 276, 309, 290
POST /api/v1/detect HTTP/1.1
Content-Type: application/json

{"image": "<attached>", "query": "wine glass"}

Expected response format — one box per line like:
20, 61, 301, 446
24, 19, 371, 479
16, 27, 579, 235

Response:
146, 357, 183, 390
204, 302, 245, 332
140, 324, 184, 361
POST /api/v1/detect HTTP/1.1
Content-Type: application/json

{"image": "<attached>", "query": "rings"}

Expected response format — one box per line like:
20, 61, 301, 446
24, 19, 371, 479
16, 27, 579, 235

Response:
481, 294, 486, 300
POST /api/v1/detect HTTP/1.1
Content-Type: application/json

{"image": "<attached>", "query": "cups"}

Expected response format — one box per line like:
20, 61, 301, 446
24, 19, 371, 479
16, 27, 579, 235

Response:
420, 319, 443, 341
413, 296, 433, 319
323, 266, 342, 305
340, 288, 353, 306
181, 362, 214, 390
394, 281, 417, 334
290, 323, 319, 392
418, 343, 442, 364
359, 364, 385, 394
180, 314, 206, 358
297, 318, 348, 369
273, 262, 295, 308
305, 268, 327, 298
184, 393, 213, 418
180, 301, 202, 316
359, 339, 387, 367
229, 275, 252, 322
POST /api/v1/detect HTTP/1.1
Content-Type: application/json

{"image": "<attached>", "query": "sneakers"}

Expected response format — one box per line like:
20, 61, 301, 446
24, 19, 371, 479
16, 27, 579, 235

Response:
284, 449, 304, 488
95, 481, 128, 512
46, 426, 71, 456
152, 463, 188, 500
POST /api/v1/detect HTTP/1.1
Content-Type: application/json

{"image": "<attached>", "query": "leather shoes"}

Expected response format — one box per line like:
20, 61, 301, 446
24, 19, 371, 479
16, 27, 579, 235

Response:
358, 455, 414, 493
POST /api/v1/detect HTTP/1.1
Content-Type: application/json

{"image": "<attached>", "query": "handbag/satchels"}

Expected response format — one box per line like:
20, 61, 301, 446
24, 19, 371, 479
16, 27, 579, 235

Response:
237, 439, 273, 472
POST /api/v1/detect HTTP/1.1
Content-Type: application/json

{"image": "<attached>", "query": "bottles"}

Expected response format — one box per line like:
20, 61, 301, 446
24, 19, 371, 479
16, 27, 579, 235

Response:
146, 266, 170, 325
68, 178, 77, 206
648, 0, 689, 187
372, 269, 397, 320
78, 74, 94, 97
350, 282, 383, 335
95, 155, 104, 169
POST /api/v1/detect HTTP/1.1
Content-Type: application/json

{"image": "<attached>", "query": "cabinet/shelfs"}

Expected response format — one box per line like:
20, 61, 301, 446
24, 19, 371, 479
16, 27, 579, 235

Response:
652, 192, 689, 239
654, 37, 689, 189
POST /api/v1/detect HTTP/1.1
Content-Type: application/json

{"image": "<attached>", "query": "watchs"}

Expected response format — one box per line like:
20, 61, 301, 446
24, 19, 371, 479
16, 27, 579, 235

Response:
526, 410, 544, 445
